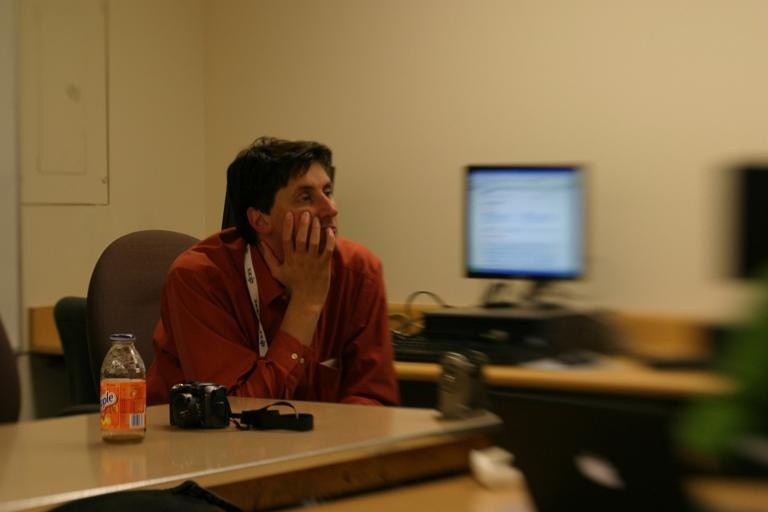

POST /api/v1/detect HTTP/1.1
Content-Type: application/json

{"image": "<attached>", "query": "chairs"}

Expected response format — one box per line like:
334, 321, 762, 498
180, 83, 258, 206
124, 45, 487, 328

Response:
52, 228, 203, 415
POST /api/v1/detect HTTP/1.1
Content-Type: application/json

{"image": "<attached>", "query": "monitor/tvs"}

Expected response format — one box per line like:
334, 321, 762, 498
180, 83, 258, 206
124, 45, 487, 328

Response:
462, 163, 589, 310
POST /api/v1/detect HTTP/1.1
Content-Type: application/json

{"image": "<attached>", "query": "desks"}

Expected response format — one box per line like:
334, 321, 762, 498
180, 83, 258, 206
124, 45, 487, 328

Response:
1, 303, 768, 512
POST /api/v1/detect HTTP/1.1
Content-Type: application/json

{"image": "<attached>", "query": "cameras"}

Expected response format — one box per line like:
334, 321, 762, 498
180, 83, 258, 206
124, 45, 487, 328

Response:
170, 382, 229, 428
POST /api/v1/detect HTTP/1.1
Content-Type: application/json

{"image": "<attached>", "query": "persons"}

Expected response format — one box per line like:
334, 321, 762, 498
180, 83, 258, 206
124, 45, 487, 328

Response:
144, 135, 403, 404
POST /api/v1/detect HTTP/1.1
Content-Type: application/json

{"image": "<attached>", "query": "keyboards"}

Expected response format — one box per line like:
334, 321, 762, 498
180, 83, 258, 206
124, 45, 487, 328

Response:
393, 335, 474, 364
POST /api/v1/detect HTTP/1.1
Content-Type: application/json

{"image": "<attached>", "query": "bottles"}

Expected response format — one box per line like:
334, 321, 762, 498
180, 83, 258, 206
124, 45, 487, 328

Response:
99, 334, 147, 444
